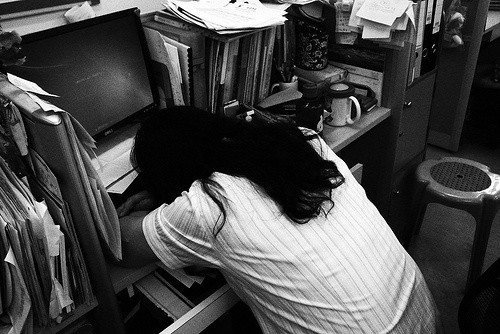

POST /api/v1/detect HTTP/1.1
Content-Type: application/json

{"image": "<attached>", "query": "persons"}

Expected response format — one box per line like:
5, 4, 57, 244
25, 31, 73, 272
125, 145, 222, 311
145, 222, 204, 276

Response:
104, 105, 443, 334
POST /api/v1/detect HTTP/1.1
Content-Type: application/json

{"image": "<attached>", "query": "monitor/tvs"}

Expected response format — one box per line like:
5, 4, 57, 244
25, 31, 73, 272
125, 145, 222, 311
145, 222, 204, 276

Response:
0, 6, 160, 142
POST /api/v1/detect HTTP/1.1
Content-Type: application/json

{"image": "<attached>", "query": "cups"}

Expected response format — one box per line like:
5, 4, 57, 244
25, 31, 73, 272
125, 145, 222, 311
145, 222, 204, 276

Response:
326, 83, 361, 127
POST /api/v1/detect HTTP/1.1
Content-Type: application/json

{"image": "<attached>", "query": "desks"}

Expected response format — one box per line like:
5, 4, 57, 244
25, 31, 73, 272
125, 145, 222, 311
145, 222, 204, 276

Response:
481, 11, 500, 41
43, 106, 391, 334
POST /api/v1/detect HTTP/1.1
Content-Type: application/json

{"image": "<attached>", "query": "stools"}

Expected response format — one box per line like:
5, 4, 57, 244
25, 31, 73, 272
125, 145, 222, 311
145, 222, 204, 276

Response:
400, 156, 500, 292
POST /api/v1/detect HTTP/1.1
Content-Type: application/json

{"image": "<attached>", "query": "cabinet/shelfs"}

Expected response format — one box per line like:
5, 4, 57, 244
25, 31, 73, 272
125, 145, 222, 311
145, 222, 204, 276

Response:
393, 69, 438, 180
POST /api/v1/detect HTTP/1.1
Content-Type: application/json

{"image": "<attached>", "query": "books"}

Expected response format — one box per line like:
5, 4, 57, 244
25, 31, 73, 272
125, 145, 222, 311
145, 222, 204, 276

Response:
0, 1, 444, 334
